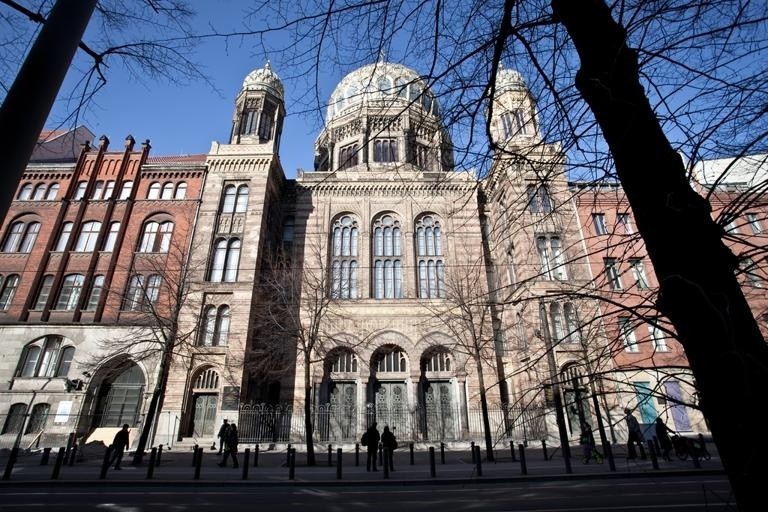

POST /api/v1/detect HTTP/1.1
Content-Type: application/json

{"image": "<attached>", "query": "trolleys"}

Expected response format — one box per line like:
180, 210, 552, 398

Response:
671, 432, 711, 462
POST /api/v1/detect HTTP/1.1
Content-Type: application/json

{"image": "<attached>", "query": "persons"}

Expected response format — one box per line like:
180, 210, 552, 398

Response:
380, 425, 396, 479
578, 423, 604, 465
360, 422, 381, 473
217, 423, 240, 469
623, 409, 646, 462
214, 418, 230, 456
107, 423, 130, 470
653, 418, 677, 462
